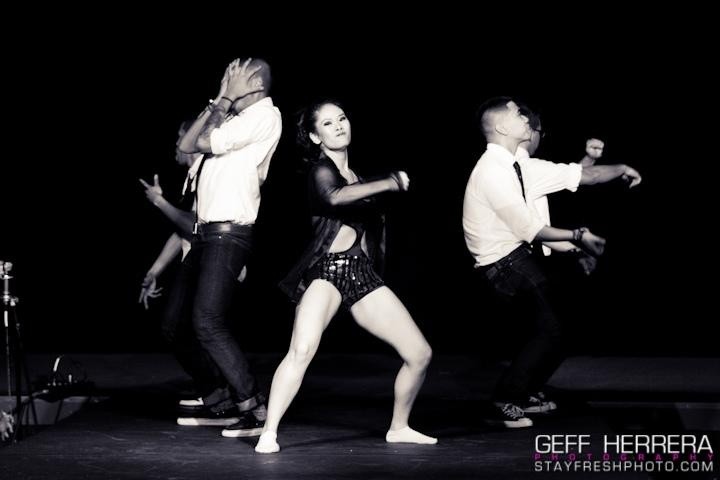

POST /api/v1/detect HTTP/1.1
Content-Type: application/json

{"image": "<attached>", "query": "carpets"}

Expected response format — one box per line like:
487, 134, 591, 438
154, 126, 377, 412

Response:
183, 173, 195, 211
514, 162, 525, 199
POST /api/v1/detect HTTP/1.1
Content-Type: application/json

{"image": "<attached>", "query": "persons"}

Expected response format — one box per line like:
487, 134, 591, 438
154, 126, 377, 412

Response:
460, 98, 642, 427
176, 56, 284, 438
0, 408, 15, 444
252, 100, 442, 453
521, 102, 606, 408
137, 120, 208, 410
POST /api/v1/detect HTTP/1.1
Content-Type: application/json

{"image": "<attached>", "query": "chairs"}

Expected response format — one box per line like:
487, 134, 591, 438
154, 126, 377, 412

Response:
485, 392, 557, 428
177, 386, 262, 438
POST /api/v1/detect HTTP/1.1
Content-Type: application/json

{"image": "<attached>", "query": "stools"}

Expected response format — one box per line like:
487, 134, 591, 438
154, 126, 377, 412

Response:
192, 222, 251, 235
486, 245, 525, 280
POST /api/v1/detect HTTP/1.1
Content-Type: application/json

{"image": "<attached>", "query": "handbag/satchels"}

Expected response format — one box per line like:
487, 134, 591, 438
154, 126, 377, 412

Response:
221, 96, 236, 105
573, 228, 581, 240
